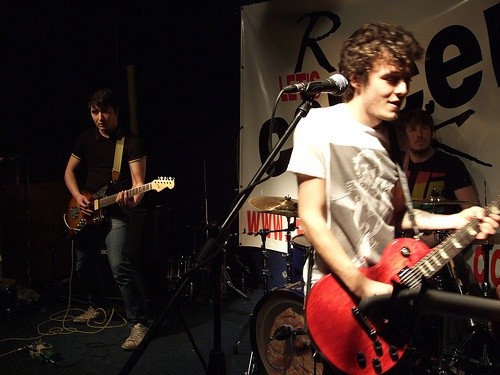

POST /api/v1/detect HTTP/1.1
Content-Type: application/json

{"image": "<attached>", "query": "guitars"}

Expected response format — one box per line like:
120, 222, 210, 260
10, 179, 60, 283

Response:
65, 177, 175, 233
307, 199, 496, 374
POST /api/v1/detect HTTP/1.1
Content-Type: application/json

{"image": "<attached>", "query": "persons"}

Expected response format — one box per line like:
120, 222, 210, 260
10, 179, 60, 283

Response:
388, 104, 483, 296
287, 23, 500, 374
62, 88, 153, 351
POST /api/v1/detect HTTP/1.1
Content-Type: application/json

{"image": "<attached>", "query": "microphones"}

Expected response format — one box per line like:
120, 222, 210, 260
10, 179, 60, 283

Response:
283, 73, 348, 96
271, 325, 293, 341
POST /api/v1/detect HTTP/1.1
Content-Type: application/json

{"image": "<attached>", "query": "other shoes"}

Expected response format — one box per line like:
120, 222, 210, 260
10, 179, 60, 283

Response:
72, 305, 110, 323
121, 323, 150, 350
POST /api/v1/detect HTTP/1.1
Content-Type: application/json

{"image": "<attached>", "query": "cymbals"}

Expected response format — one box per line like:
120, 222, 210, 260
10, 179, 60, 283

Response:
249, 196, 297, 217
405, 195, 478, 206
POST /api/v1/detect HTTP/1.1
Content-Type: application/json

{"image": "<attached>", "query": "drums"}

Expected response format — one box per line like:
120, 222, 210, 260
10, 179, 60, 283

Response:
253, 283, 327, 375
291, 234, 313, 267
